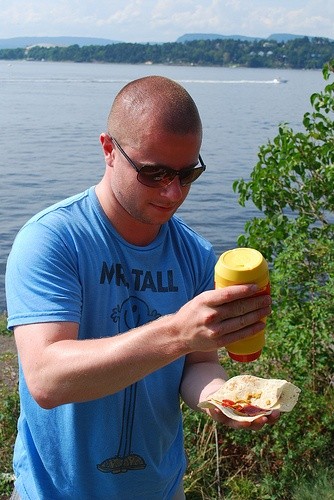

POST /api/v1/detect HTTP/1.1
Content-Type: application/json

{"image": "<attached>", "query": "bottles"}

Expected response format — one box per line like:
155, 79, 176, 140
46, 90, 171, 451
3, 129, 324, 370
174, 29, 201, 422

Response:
214, 248, 270, 362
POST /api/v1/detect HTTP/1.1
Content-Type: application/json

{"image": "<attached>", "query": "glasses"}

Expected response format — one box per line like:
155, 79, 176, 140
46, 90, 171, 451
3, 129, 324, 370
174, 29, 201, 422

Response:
112, 136, 206, 190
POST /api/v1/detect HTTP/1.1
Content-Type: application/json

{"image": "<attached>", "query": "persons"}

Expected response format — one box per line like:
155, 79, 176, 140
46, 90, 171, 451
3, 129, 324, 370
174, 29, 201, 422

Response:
5, 76, 281, 500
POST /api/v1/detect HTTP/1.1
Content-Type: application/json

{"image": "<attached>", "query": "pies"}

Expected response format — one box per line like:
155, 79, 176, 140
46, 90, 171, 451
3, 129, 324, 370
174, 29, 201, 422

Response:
197, 374, 302, 422
